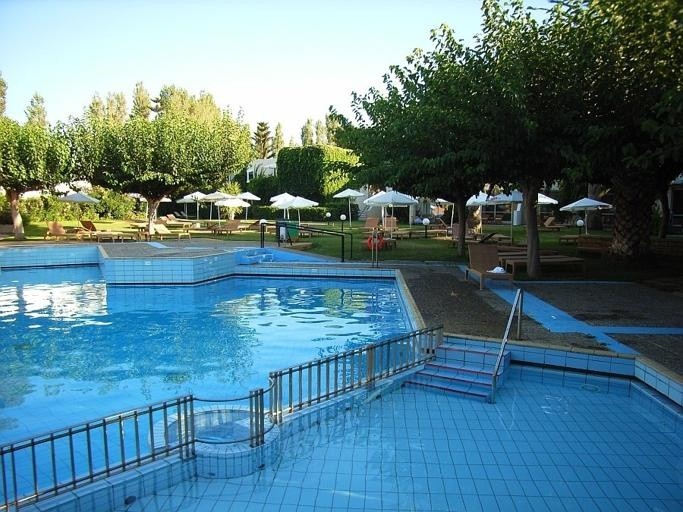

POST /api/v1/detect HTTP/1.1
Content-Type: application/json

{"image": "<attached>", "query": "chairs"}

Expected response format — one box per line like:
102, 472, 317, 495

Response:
45, 211, 240, 242
464, 217, 601, 290
359, 216, 448, 251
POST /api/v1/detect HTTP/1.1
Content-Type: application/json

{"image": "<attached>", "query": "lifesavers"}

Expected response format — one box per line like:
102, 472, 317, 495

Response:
368, 236, 384, 250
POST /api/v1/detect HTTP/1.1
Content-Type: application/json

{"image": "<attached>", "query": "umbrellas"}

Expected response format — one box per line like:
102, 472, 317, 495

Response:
436, 199, 457, 230
559, 197, 614, 233
55, 189, 102, 231
489, 187, 559, 245
468, 191, 495, 233
174, 188, 419, 230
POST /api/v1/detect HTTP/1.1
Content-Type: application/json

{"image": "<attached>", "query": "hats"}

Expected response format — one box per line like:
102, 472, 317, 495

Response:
486, 266, 506, 274
